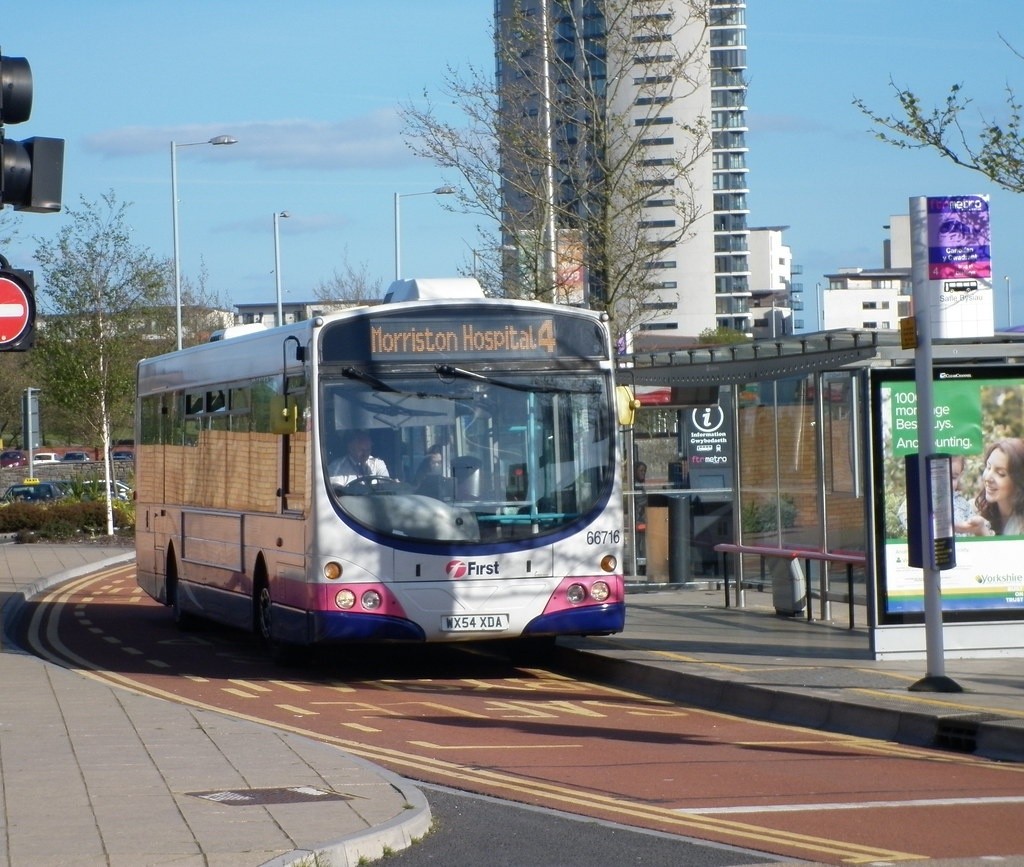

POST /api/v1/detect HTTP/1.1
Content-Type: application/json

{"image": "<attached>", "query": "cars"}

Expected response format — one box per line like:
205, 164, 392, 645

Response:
0, 479, 132, 503
0, 451, 132, 469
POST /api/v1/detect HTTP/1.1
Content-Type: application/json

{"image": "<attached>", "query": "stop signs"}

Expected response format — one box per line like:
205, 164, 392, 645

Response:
0, 276, 29, 348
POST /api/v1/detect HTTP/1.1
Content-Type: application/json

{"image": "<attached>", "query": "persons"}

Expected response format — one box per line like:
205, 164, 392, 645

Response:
327, 429, 389, 487
427, 444, 442, 473
897, 453, 992, 537
633, 461, 647, 576
966, 438, 1024, 537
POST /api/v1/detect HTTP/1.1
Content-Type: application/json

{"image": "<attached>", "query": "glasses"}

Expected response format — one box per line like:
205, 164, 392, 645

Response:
639, 469, 646, 472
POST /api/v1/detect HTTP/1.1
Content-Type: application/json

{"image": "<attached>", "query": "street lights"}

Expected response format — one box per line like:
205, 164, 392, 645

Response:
395, 185, 458, 280
773, 282, 822, 336
1006, 276, 1012, 328
23, 387, 41, 479
473, 246, 517, 277
171, 135, 239, 350
274, 211, 292, 327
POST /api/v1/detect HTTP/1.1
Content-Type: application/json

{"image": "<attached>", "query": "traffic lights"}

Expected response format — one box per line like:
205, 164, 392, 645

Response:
508, 464, 527, 501
0, 53, 66, 217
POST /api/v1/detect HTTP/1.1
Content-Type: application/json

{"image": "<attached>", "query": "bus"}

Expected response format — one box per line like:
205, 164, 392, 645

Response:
133, 277, 642, 660
